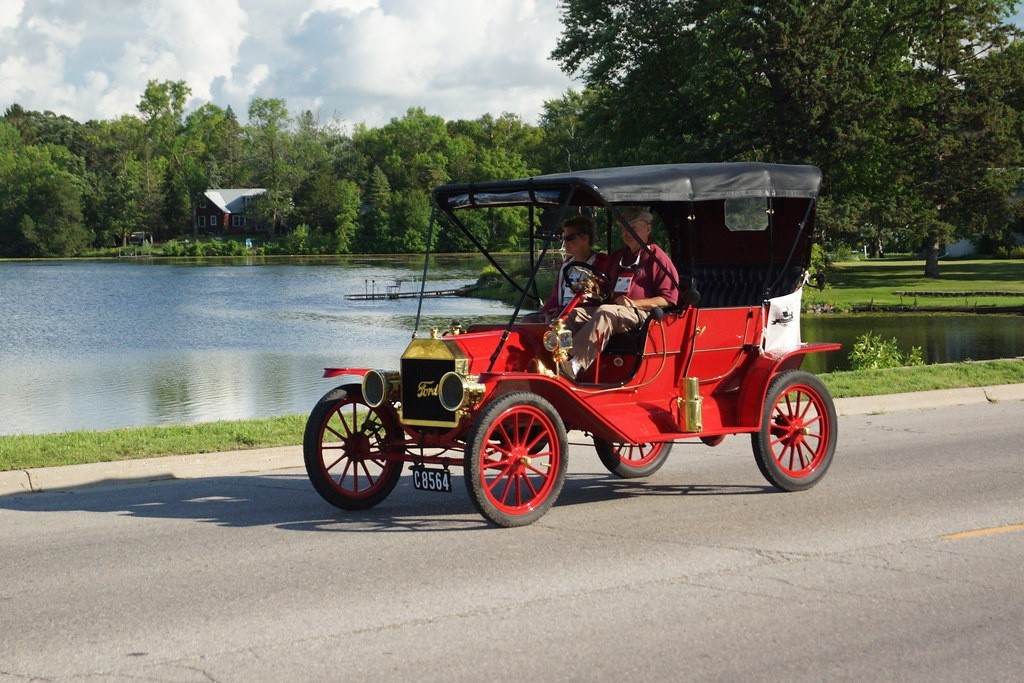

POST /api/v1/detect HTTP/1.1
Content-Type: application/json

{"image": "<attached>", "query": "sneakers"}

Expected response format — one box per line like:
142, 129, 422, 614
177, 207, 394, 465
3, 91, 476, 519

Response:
559, 360, 578, 383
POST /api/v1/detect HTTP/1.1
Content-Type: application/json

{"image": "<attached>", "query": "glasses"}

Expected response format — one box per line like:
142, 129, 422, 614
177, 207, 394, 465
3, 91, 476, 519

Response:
562, 232, 583, 241
617, 248, 641, 270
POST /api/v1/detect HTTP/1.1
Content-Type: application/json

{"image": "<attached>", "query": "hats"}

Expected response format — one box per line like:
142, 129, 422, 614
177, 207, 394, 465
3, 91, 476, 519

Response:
616, 208, 653, 224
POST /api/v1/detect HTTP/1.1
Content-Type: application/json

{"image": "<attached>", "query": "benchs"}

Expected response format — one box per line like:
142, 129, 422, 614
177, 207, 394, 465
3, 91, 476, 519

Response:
675, 261, 795, 308
601, 274, 700, 354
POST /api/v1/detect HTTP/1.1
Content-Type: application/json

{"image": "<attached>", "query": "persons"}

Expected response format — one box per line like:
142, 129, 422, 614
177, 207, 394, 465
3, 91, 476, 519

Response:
518, 215, 608, 324
559, 208, 680, 382
